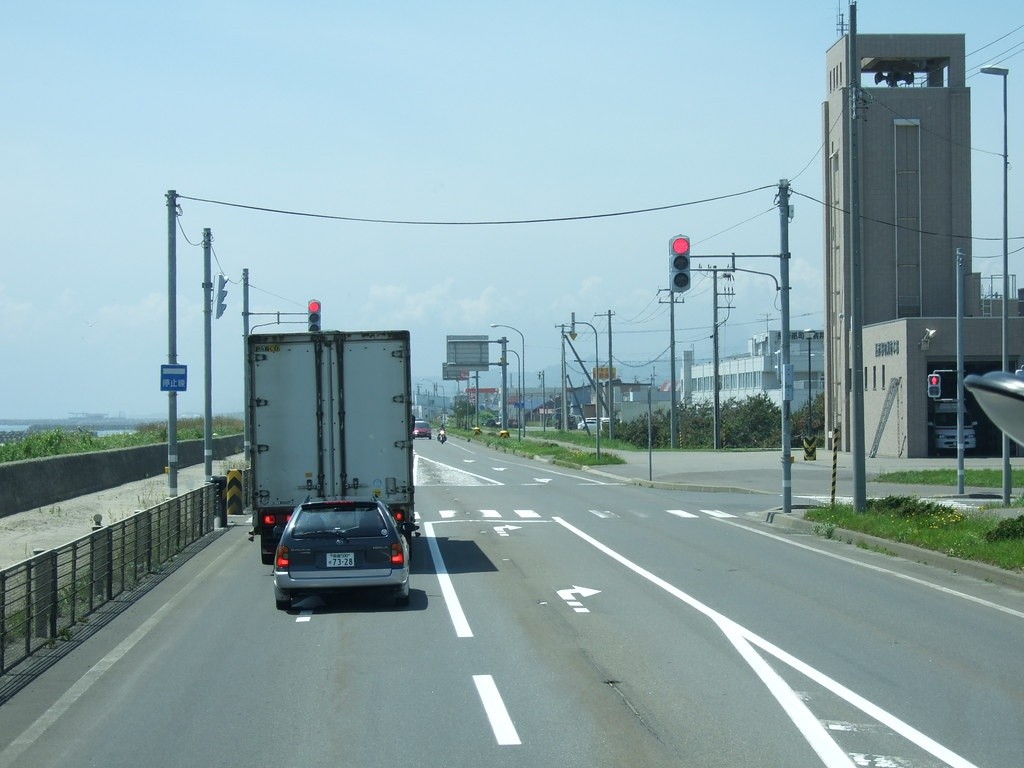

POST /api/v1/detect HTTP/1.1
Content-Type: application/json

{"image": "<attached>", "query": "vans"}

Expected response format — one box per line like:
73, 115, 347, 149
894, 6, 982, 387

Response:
928, 397, 979, 452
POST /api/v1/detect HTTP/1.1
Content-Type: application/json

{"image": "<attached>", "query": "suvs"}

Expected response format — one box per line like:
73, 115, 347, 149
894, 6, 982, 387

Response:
412, 421, 432, 440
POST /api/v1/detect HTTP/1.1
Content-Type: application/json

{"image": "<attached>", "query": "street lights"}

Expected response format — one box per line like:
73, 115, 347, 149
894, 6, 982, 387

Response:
980, 65, 1012, 505
572, 322, 600, 460
490, 324, 526, 438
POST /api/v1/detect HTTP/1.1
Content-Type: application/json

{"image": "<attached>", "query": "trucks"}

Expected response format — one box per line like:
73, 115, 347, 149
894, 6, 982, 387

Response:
247, 330, 419, 561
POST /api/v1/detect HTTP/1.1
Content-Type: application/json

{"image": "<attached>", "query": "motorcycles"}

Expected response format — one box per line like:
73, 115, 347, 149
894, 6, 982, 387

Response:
437, 429, 446, 444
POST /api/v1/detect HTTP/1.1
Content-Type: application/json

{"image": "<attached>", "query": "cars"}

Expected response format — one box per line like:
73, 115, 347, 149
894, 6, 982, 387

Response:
272, 495, 411, 609
486, 419, 501, 428
577, 418, 596, 430
508, 419, 519, 428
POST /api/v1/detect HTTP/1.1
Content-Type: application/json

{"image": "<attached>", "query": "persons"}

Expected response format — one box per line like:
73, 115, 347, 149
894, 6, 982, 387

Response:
437, 423, 447, 441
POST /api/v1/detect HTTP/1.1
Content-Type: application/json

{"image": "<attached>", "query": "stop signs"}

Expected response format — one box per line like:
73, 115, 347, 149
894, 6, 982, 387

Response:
160, 365, 187, 391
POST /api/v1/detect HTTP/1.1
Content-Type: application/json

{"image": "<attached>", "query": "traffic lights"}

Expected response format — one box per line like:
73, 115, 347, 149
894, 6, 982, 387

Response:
668, 235, 692, 293
928, 374, 941, 397
309, 299, 322, 331
